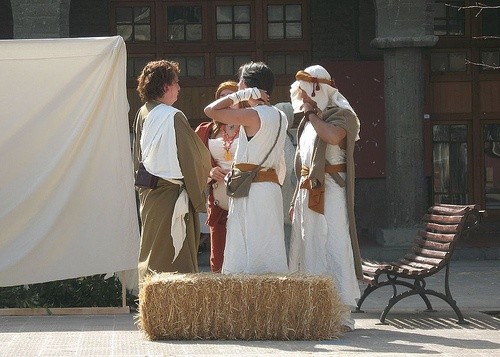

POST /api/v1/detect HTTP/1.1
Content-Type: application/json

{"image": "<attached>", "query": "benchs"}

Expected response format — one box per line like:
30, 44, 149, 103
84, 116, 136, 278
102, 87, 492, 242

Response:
350, 202, 489, 325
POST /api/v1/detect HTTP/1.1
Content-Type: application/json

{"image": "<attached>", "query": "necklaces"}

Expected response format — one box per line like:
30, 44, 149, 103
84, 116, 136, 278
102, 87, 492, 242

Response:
219, 123, 241, 161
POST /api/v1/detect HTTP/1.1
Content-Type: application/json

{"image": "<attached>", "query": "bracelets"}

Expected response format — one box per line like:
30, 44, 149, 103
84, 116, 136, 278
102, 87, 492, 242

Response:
304, 110, 317, 121
290, 201, 294, 206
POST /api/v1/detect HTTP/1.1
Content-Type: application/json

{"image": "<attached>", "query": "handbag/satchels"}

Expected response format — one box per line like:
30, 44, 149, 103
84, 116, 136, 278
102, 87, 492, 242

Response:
223, 168, 255, 198
135, 163, 158, 190
212, 180, 229, 211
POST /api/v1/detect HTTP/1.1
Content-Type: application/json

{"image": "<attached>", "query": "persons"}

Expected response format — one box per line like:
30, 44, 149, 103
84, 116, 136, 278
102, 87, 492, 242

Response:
204, 61, 290, 276
131, 59, 212, 307
288, 64, 364, 333
194, 81, 249, 275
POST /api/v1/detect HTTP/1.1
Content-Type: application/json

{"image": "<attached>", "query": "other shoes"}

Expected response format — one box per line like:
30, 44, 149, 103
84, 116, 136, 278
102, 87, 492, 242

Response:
340, 318, 355, 332
